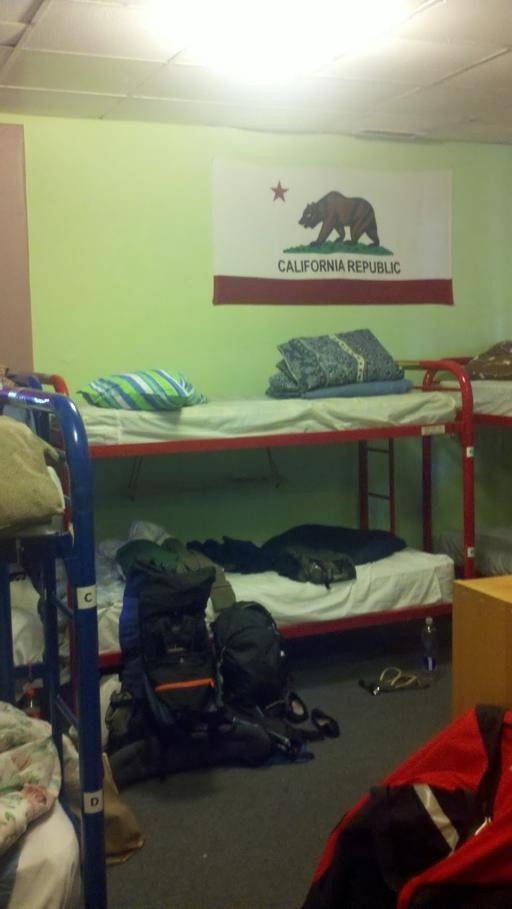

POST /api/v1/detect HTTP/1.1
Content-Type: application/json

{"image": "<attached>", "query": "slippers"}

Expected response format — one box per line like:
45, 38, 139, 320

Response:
359, 666, 421, 691
370, 674, 434, 694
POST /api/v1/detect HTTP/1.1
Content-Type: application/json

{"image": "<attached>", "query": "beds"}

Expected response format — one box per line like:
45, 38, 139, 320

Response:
424, 357, 512, 575
0, 388, 106, 909
18, 361, 476, 680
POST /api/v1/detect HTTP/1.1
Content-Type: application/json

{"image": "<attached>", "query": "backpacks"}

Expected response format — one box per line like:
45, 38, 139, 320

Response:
207, 599, 291, 713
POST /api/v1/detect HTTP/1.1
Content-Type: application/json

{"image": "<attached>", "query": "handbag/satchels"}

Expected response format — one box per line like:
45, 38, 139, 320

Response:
290, 543, 358, 582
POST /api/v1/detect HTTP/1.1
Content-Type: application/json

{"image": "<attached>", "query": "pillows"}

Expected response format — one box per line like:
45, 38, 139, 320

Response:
79, 369, 207, 407
455, 340, 512, 379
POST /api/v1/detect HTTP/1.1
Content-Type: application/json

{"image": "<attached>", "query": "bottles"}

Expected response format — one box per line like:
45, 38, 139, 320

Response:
421, 621, 440, 675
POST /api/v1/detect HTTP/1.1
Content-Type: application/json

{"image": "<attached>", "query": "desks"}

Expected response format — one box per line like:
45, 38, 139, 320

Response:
451, 574, 512, 709
